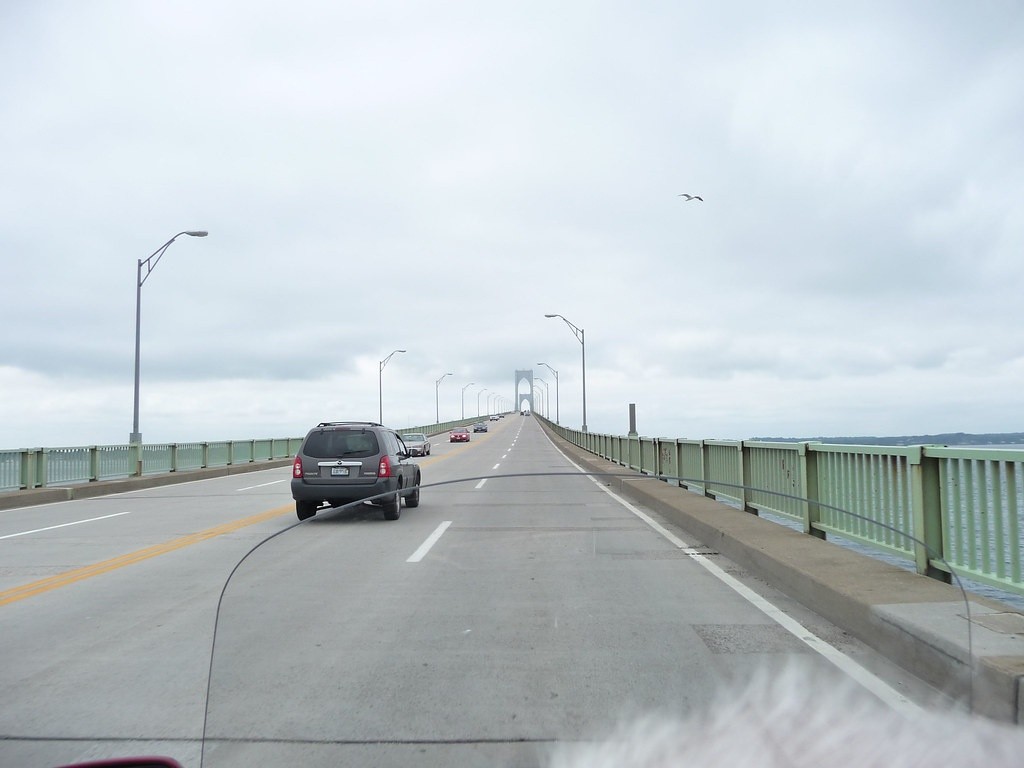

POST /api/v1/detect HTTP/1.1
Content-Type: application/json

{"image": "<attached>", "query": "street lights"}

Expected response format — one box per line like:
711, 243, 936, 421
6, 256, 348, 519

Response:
544, 313, 587, 432
378, 349, 406, 424
533, 384, 544, 418
130, 230, 209, 441
477, 387, 488, 416
436, 373, 454, 423
493, 394, 511, 415
536, 362, 559, 425
461, 382, 476, 420
487, 391, 497, 414
534, 376, 549, 419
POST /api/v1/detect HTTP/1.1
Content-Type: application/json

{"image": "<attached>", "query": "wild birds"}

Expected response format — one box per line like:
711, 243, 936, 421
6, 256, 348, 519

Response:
677, 193, 704, 202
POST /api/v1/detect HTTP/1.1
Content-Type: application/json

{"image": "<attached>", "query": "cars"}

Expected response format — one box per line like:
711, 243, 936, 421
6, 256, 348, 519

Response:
401, 433, 430, 456
520, 412, 524, 415
490, 415, 499, 421
498, 414, 504, 418
526, 412, 530, 416
510, 410, 515, 414
449, 428, 471, 442
473, 422, 487, 432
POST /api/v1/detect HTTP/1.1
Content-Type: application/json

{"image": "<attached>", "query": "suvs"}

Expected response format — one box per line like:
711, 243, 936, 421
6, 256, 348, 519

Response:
292, 420, 421, 522
